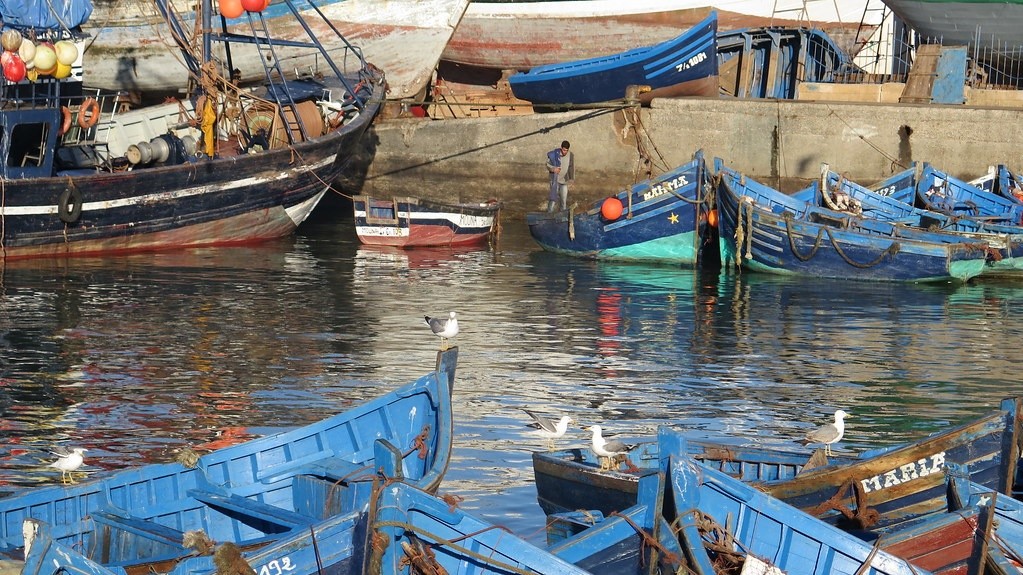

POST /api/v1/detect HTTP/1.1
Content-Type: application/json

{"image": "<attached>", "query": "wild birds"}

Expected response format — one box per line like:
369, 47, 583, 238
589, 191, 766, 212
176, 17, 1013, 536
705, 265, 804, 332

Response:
584, 426, 637, 472
520, 408, 575, 453
39, 439, 89, 486
793, 410, 851, 457
423, 312, 460, 351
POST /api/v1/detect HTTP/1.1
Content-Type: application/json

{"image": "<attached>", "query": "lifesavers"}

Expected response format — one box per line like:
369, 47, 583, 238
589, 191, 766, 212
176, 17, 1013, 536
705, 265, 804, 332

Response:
59, 304, 79, 328
58, 188, 82, 223
78, 98, 99, 129
58, 106, 72, 136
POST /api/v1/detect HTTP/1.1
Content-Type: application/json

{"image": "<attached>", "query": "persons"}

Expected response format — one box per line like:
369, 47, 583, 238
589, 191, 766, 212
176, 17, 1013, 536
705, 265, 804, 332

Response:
546, 141, 575, 213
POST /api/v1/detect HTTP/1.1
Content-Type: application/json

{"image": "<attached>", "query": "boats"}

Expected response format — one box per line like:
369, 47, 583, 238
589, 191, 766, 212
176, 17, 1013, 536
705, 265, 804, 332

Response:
508, 13, 723, 115
0, 344, 464, 575
52, 1, 882, 101
352, 193, 500, 248
1, 394, 1023, 575
528, 148, 1023, 284
0, 0, 391, 261
879, 0, 1022, 62
